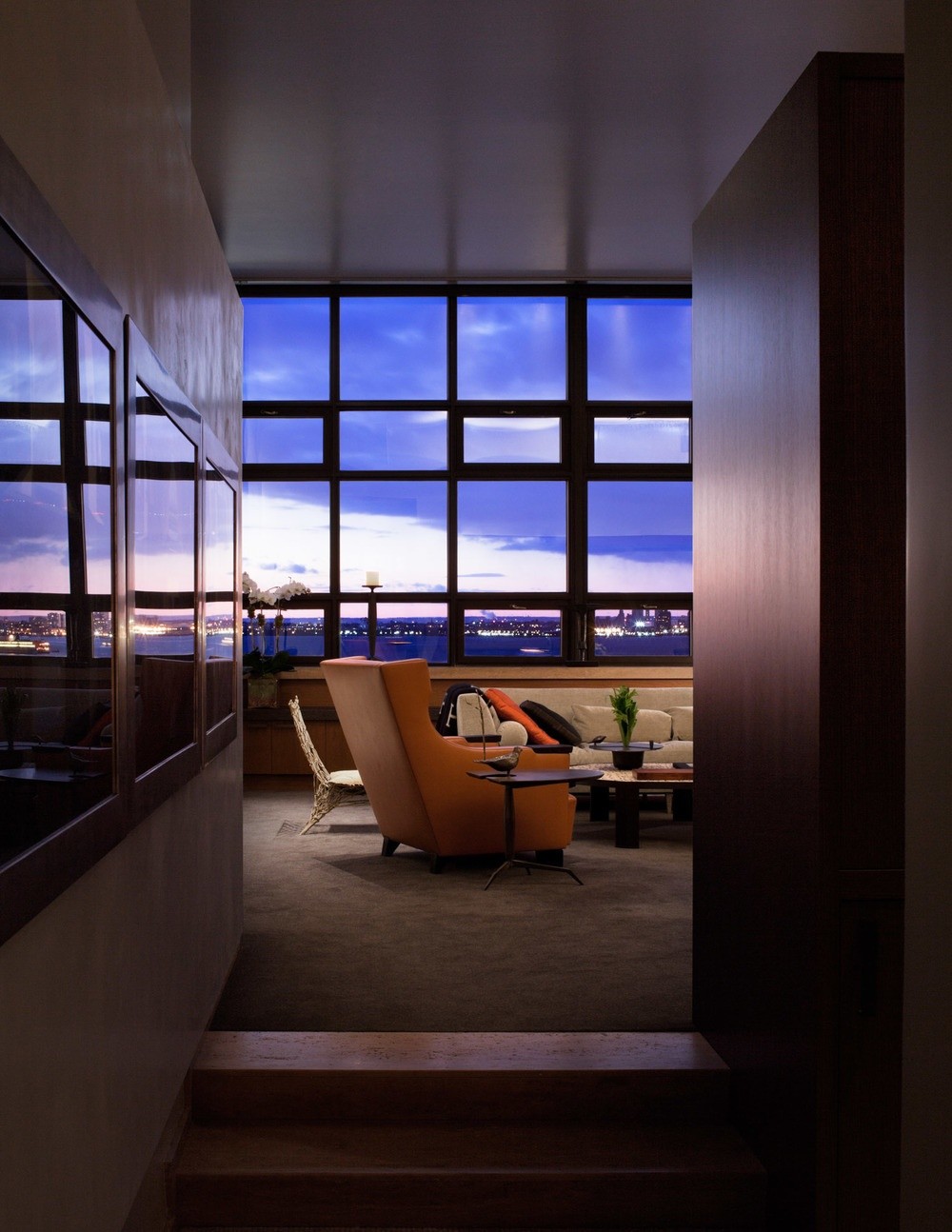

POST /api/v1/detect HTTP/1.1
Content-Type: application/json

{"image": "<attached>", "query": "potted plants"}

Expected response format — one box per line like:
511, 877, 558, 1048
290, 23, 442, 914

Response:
609, 687, 640, 770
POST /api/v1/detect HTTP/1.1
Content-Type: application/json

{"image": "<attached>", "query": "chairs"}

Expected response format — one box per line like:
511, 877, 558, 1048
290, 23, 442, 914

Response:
289, 697, 366, 839
135, 655, 232, 778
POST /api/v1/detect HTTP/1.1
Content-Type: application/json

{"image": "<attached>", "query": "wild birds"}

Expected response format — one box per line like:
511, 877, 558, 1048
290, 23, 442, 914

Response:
473, 747, 523, 780
587, 735, 607, 747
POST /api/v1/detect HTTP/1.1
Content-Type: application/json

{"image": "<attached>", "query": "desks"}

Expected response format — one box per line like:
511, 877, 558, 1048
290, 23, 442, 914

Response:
568, 763, 692, 847
0, 762, 108, 815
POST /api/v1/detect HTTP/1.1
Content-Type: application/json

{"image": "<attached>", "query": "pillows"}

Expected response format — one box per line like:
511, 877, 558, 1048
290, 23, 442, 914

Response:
18, 706, 64, 742
631, 708, 677, 741
563, 700, 629, 745
668, 706, 693, 741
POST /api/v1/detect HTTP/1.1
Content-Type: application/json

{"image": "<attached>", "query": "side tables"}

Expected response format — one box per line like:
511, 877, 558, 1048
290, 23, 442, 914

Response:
465, 770, 605, 889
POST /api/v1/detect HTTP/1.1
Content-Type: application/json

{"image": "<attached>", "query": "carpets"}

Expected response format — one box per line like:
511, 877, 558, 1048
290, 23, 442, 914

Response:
205, 790, 695, 1032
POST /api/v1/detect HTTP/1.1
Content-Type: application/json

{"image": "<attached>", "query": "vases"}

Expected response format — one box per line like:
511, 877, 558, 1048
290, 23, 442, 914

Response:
249, 673, 280, 708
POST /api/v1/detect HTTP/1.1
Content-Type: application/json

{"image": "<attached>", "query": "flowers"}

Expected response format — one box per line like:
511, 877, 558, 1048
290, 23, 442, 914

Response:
242, 572, 311, 679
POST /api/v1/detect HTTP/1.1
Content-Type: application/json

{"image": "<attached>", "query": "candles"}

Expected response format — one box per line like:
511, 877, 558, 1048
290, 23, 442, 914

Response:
366, 572, 379, 585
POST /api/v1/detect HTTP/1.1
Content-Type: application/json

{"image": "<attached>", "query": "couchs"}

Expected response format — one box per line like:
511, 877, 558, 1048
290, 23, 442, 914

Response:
320, 656, 576, 873
0, 687, 142, 747
451, 687, 697, 825
